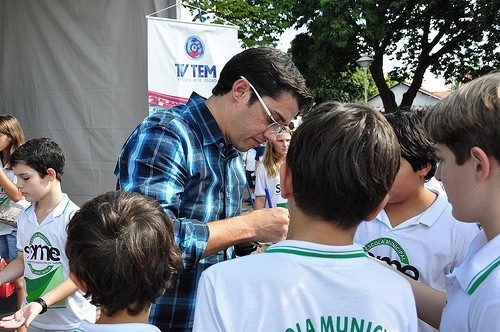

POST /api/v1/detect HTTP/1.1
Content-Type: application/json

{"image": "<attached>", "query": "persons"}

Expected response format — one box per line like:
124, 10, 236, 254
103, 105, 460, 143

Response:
191, 102, 418, 331
0, 137, 96, 332
366, 71, 499, 331
243, 122, 295, 211
113, 47, 313, 332
353, 109, 480, 331
0, 114, 28, 332
69, 190, 185, 332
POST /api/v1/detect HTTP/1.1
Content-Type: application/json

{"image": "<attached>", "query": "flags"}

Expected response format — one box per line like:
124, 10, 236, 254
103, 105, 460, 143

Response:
146, 16, 237, 117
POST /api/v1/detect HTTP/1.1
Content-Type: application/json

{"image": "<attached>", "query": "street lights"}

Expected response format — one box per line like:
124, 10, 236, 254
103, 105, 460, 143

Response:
356, 54, 375, 104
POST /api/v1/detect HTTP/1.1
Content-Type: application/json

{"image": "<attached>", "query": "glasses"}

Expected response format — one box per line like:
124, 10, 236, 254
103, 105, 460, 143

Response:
240, 76, 283, 135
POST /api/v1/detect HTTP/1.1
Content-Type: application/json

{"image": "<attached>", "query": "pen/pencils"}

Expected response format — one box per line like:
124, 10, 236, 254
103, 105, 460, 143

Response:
264, 188, 272, 208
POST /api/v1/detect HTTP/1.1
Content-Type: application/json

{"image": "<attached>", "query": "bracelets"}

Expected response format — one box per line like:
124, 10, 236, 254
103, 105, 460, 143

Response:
33, 298, 47, 315
